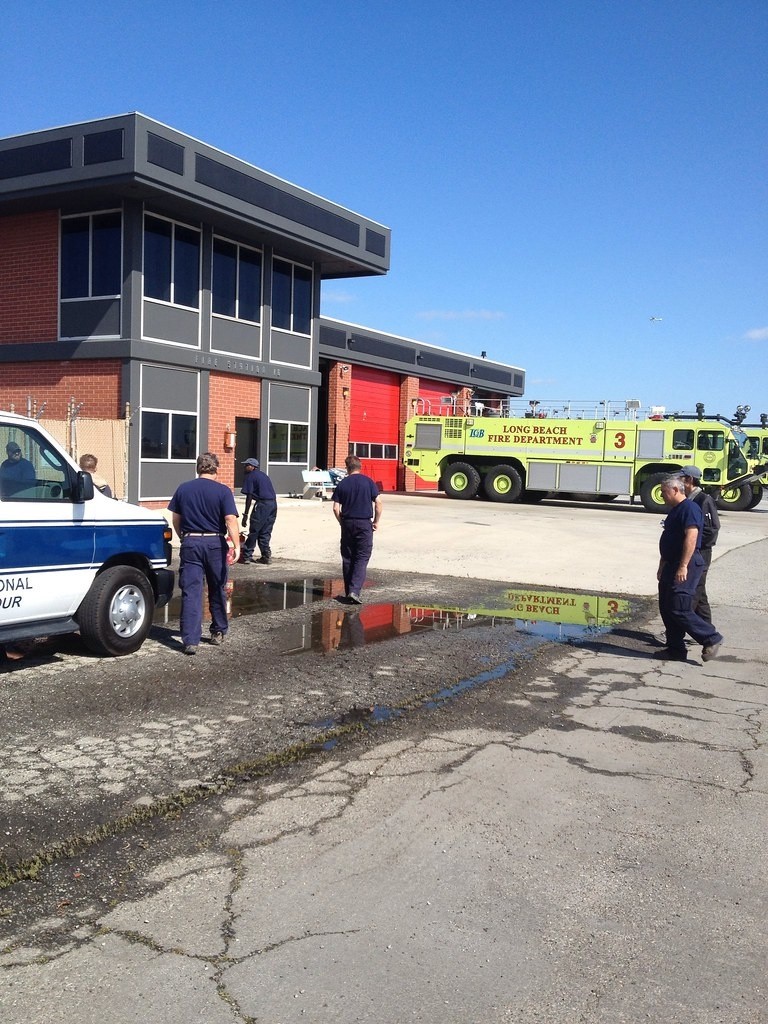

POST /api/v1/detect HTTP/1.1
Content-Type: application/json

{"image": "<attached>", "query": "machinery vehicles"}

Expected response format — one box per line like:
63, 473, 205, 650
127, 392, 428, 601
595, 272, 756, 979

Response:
403, 393, 768, 514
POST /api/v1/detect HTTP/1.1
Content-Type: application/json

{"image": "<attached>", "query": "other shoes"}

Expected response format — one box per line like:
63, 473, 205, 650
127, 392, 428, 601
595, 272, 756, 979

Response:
256, 558, 272, 564
654, 632, 667, 644
245, 560, 250, 564
689, 639, 699, 645
211, 632, 224, 644
653, 648, 688, 660
346, 592, 362, 604
701, 636, 725, 662
185, 645, 197, 653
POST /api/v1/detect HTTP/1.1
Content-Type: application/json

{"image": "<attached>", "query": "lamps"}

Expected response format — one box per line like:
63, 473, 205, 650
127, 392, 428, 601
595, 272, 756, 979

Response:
224, 431, 236, 449
343, 387, 349, 396
412, 398, 418, 407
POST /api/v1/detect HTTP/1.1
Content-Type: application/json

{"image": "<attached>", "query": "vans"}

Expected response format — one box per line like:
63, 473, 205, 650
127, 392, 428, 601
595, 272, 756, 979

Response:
0, 407, 177, 658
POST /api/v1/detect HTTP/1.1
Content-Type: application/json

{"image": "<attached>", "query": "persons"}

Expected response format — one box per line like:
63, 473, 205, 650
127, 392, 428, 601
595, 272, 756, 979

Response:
651, 466, 723, 661
78, 454, 112, 499
241, 458, 277, 565
332, 456, 383, 604
166, 452, 240, 654
0, 442, 36, 480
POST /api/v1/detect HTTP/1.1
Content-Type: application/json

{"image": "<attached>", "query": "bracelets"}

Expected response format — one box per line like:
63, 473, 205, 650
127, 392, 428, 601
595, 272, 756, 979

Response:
242, 513, 248, 516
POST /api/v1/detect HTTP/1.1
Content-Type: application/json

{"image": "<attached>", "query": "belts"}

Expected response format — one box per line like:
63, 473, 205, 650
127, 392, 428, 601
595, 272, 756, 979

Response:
184, 533, 224, 537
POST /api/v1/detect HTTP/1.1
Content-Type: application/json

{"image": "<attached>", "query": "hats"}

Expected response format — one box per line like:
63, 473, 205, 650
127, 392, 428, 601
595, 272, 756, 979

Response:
6, 442, 21, 453
671, 465, 702, 479
241, 458, 259, 467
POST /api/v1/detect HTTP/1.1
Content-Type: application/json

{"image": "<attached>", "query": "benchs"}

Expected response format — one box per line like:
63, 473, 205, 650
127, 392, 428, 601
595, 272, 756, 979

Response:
302, 470, 337, 502
